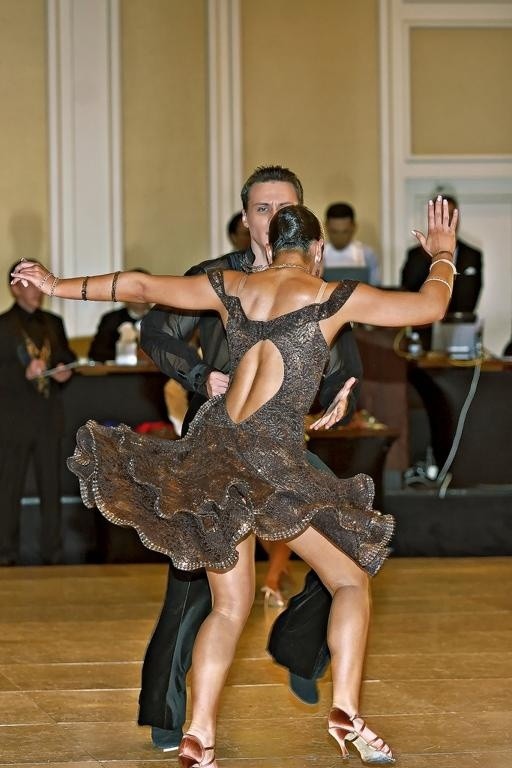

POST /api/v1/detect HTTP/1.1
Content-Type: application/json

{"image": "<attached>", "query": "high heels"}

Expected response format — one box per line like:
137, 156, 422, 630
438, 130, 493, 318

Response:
328, 706, 395, 765
179, 734, 217, 768
261, 586, 285, 607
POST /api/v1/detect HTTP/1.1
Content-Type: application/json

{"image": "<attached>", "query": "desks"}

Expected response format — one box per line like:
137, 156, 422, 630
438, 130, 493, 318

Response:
20, 335, 512, 566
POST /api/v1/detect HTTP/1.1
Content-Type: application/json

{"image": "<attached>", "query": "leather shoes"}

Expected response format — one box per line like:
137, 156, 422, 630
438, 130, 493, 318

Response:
290, 673, 319, 705
151, 725, 183, 752
